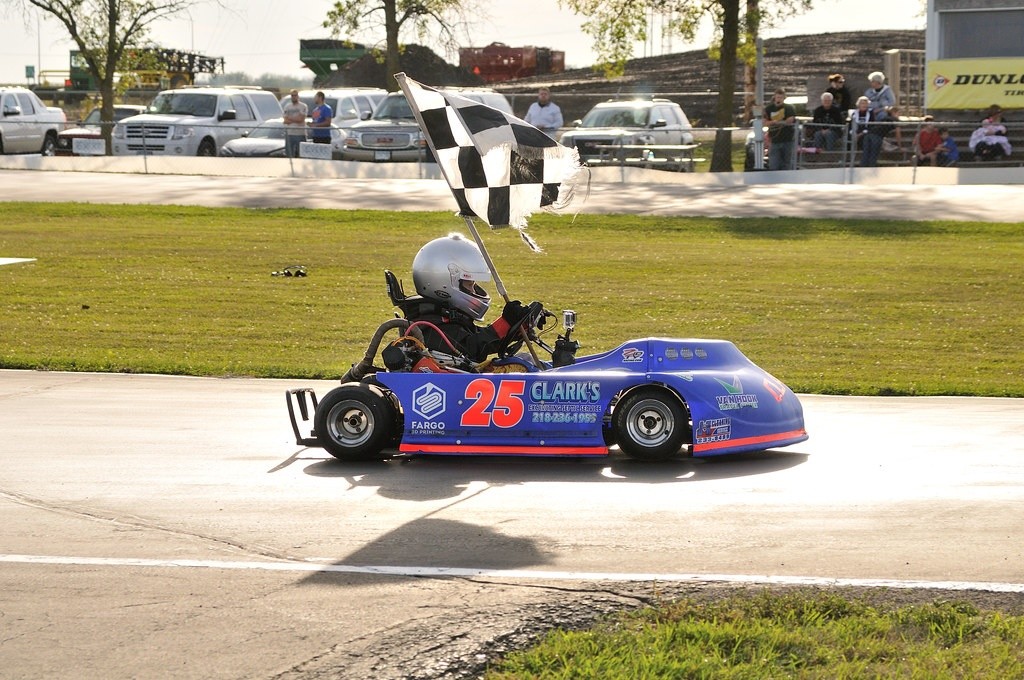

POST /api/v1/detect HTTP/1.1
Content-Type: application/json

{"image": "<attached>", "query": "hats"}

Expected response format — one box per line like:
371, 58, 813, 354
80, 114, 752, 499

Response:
923, 114, 934, 121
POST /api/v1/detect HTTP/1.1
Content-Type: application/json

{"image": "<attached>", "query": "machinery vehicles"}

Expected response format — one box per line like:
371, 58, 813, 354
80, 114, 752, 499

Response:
65, 47, 225, 91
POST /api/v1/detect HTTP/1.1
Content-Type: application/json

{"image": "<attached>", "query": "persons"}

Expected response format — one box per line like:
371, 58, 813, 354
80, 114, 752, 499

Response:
911, 117, 959, 167
825, 74, 851, 123
969, 104, 1012, 162
525, 87, 563, 140
404, 234, 552, 373
813, 92, 843, 150
763, 89, 795, 171
310, 92, 332, 144
283, 89, 308, 158
849, 72, 902, 167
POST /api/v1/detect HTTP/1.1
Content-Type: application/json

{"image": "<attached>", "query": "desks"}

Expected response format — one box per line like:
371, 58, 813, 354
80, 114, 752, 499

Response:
594, 144, 697, 171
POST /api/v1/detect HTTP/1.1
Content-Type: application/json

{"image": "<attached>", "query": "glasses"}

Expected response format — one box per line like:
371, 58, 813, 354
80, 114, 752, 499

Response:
997, 110, 1003, 115
839, 80, 847, 84
938, 128, 947, 134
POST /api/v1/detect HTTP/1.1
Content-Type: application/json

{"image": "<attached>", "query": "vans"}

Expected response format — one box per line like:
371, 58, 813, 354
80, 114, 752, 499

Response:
279, 87, 388, 136
111, 88, 284, 157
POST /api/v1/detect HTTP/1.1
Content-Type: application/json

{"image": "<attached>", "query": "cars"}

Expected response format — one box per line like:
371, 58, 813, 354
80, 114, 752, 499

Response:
744, 116, 815, 172
0, 88, 67, 156
54, 105, 156, 156
219, 117, 349, 160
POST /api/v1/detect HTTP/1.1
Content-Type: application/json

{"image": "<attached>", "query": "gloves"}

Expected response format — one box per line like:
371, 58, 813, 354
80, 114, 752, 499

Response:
502, 300, 530, 327
537, 313, 550, 330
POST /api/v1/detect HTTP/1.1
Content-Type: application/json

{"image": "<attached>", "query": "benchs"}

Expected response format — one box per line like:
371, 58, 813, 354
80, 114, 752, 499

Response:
803, 120, 1024, 167
586, 158, 706, 172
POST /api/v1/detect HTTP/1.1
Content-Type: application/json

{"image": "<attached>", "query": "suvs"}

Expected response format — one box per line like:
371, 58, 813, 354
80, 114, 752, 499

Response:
341, 86, 515, 162
559, 99, 695, 161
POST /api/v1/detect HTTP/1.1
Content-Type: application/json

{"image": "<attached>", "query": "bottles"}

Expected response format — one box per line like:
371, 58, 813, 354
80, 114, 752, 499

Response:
648, 152, 655, 161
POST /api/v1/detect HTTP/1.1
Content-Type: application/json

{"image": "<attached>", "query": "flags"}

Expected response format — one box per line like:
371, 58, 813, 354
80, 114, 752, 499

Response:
405, 77, 591, 254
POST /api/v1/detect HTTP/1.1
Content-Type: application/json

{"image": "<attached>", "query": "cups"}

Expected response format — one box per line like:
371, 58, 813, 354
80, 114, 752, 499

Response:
642, 149, 650, 161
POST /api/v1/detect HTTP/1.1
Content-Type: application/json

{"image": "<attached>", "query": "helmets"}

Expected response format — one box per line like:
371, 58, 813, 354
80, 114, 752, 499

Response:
413, 233, 492, 321
